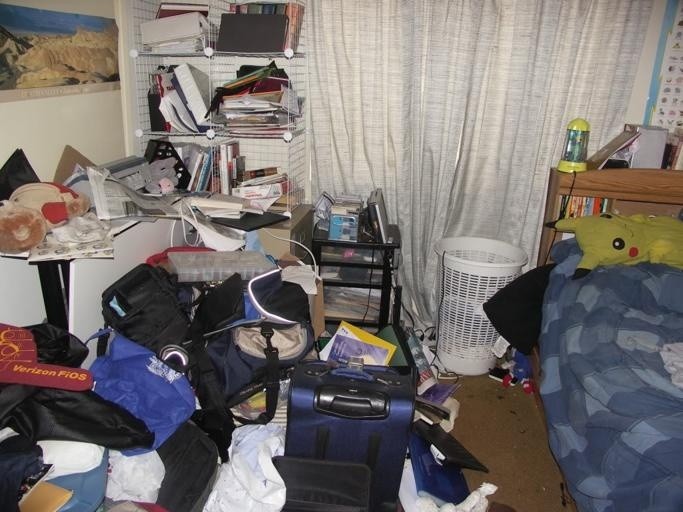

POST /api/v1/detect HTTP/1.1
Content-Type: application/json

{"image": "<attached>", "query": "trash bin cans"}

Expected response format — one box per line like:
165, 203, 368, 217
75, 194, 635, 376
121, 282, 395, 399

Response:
434, 236, 528, 376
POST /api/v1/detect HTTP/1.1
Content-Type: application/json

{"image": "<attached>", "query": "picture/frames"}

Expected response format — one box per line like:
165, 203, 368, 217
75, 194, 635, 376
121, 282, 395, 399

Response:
0, 3, 121, 104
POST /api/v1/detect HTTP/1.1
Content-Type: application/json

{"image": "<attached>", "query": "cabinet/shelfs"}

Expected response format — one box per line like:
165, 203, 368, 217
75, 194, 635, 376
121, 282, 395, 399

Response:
130, 1, 308, 219
310, 215, 401, 331
537, 170, 683, 274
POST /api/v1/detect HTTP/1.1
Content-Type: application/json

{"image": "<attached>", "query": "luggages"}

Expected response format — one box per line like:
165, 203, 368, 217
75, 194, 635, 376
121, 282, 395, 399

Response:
0, 323, 221, 511
285, 359, 416, 502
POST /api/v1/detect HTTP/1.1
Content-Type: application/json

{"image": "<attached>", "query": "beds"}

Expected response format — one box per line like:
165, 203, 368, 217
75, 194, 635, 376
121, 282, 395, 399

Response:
533, 226, 683, 511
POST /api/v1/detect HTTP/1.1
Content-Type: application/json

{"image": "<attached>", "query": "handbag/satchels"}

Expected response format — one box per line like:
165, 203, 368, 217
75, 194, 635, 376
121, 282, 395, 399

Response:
102, 250, 315, 425
80, 329, 195, 456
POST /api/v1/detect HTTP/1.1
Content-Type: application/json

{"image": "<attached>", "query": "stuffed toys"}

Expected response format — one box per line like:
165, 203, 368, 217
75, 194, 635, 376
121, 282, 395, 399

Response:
0, 182, 90, 253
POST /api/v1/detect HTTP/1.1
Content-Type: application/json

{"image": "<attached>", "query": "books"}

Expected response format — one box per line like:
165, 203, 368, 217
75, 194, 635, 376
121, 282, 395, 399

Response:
320, 266, 342, 280
216, 3, 305, 52
147, 64, 216, 134
324, 286, 382, 320
172, 142, 219, 193
328, 191, 368, 243
557, 195, 608, 219
336, 320, 397, 366
219, 141, 305, 211
189, 191, 251, 219
661, 126, 683, 170
218, 66, 304, 135
489, 366, 522, 386
321, 250, 362, 262
327, 334, 389, 366
365, 269, 383, 282
408, 433, 470, 508
230, 405, 288, 427
156, 2, 209, 19
587, 131, 641, 170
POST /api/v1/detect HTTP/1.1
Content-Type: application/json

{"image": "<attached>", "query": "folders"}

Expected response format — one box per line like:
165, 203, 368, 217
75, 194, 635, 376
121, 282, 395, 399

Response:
139, 11, 215, 45
173, 62, 223, 127
216, 12, 290, 53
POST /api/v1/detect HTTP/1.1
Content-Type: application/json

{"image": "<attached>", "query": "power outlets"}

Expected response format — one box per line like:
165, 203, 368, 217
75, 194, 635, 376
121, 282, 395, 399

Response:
418, 335, 437, 346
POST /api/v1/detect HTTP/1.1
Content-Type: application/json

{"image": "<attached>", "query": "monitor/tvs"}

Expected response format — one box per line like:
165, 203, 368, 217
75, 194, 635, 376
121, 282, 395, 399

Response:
368, 187, 390, 244
